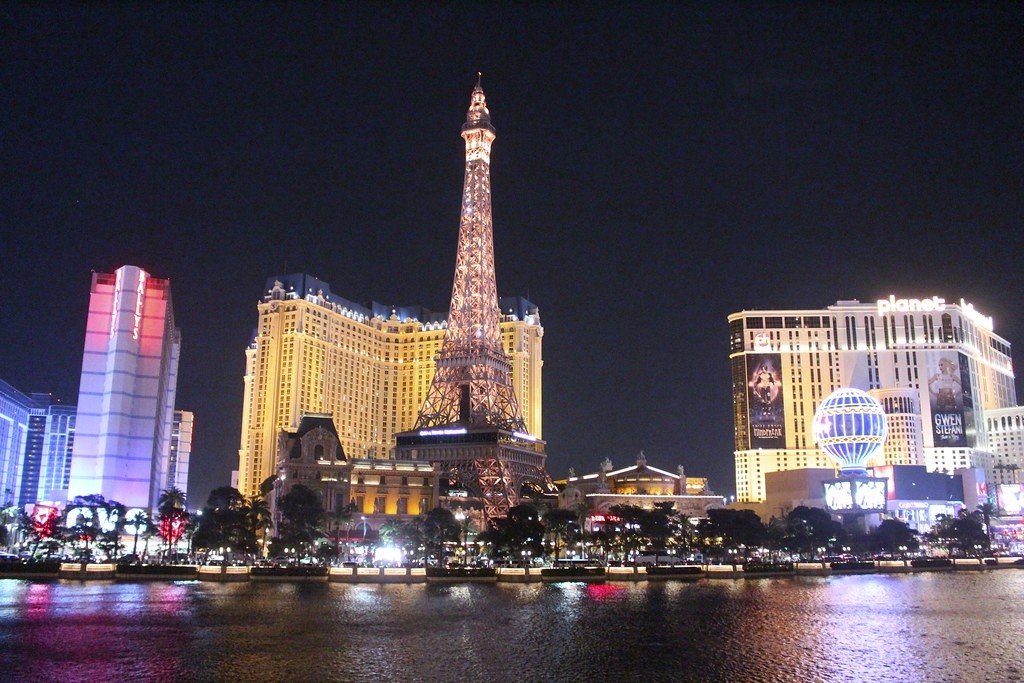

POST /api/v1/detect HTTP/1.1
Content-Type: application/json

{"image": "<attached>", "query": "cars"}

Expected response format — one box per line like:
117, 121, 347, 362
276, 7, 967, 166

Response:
252, 556, 318, 567
0, 551, 140, 564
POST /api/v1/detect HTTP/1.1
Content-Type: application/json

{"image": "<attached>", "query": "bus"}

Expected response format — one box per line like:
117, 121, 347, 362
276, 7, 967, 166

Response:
490, 560, 531, 568
635, 551, 683, 566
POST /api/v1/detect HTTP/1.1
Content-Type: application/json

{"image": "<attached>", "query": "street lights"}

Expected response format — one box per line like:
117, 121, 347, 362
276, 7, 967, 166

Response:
298, 542, 303, 567
919, 545, 927, 567
219, 546, 231, 566
817, 547, 826, 568
974, 545, 982, 564
843, 546, 850, 563
728, 549, 738, 571
283, 548, 296, 565
521, 550, 531, 575
898, 546, 908, 566
667, 549, 676, 568
630, 547, 639, 573
566, 550, 575, 565
